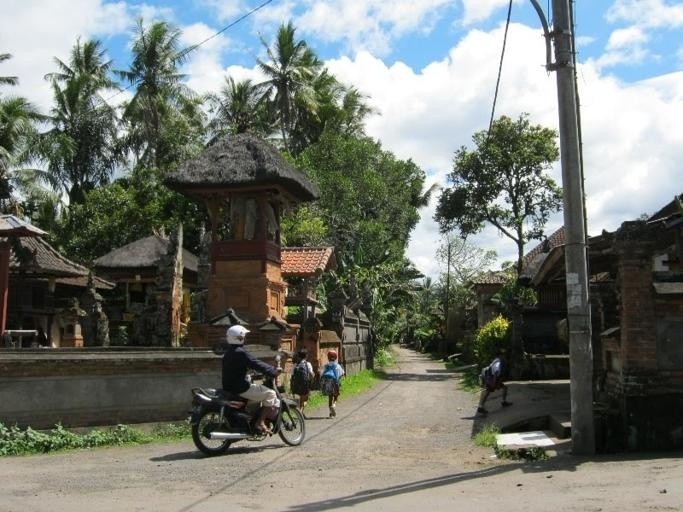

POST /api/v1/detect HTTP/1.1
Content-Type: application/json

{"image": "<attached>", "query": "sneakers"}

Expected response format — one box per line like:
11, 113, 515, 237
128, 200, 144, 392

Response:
478, 407, 488, 413
328, 405, 337, 417
501, 401, 513, 408
254, 423, 272, 436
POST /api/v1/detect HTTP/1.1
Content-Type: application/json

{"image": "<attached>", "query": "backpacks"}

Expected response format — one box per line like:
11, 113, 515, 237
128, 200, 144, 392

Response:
320, 363, 339, 398
291, 361, 310, 395
478, 365, 493, 389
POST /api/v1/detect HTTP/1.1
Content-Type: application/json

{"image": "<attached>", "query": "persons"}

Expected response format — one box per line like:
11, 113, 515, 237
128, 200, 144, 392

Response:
290, 349, 315, 420
319, 348, 344, 418
476, 348, 513, 414
220, 324, 282, 436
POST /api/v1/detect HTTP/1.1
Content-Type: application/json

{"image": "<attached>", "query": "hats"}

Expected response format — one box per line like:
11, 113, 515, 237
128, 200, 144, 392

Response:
327, 351, 336, 359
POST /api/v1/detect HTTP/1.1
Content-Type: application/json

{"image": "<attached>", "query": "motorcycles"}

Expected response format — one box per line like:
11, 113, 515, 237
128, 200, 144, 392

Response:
188, 366, 306, 454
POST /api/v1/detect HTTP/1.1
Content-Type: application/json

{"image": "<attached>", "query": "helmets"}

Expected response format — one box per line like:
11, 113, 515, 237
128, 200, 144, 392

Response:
226, 325, 250, 345
